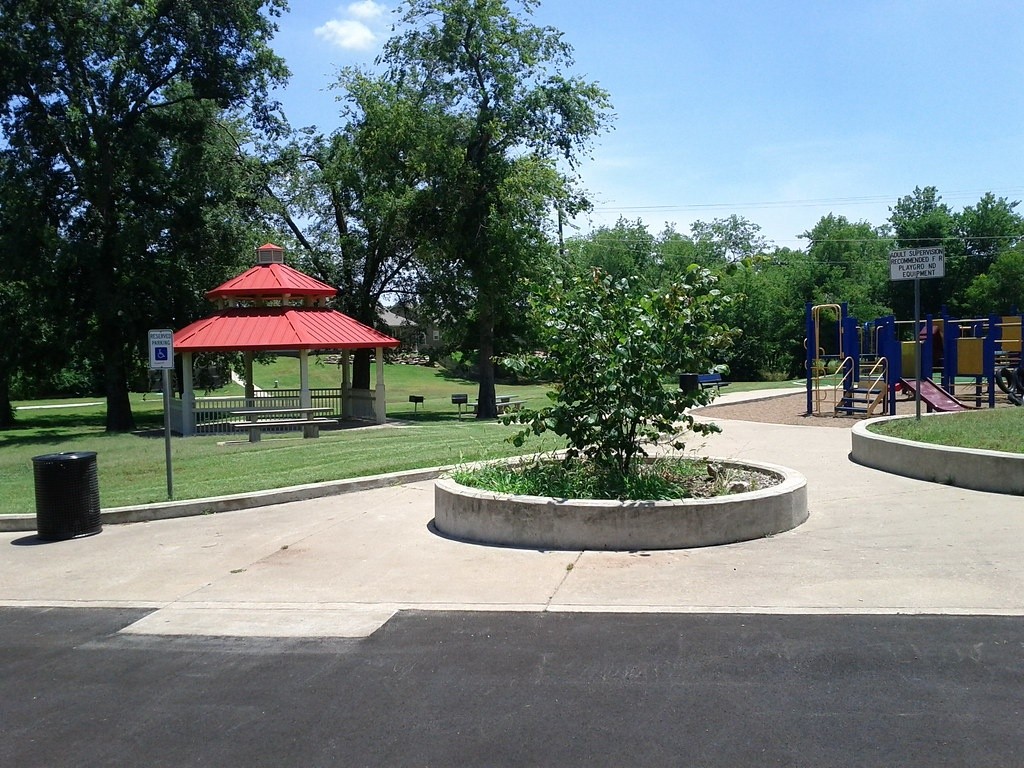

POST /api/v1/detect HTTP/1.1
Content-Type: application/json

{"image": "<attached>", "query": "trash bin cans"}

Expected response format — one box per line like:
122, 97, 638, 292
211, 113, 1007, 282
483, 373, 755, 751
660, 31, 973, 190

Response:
679, 373, 699, 395
32, 450, 102, 543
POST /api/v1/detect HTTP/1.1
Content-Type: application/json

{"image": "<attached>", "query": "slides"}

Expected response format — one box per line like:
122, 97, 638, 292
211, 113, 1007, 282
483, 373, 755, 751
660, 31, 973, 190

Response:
900, 375, 986, 412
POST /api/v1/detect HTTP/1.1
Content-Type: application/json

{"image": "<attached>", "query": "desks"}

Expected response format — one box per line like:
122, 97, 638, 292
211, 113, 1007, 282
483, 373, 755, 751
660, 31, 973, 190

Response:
230, 407, 334, 442
476, 395, 519, 412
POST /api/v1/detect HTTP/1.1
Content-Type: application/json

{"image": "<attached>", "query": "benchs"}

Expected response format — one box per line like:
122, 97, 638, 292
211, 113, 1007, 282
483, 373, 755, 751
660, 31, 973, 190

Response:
228, 417, 338, 428
467, 401, 501, 414
698, 373, 731, 392
495, 400, 527, 414
